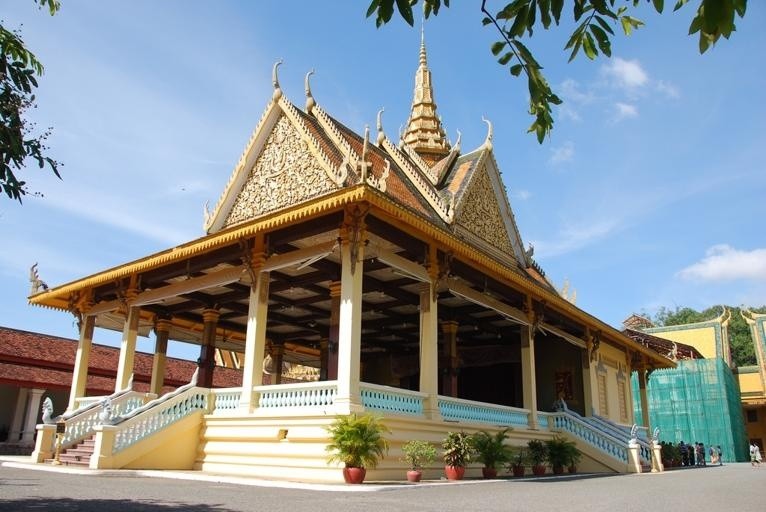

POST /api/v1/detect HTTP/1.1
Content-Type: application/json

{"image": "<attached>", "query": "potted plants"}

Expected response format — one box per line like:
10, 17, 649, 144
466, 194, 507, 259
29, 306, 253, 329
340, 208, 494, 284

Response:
662, 444, 682, 468
324, 409, 584, 484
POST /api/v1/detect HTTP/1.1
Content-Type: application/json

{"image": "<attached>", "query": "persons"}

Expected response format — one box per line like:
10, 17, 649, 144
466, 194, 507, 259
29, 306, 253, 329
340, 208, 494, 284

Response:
709, 445, 714, 463
754, 443, 763, 464
717, 446, 723, 466
658, 440, 706, 466
749, 441, 756, 466
